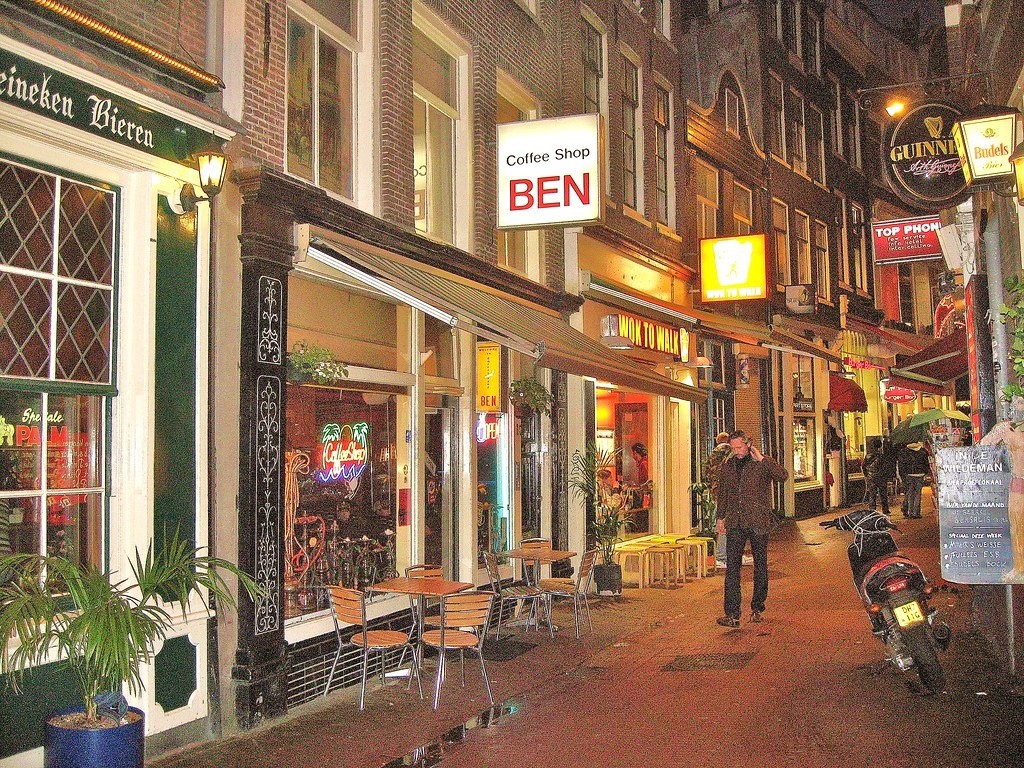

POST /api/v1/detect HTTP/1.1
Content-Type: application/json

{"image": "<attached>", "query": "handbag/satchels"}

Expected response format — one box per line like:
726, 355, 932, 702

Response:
862, 463, 868, 477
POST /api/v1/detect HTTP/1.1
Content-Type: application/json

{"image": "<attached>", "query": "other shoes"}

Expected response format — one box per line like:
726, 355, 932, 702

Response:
716, 560, 727, 569
750, 610, 764, 622
741, 555, 754, 565
901, 508, 908, 514
904, 513, 914, 519
912, 514, 922, 519
882, 510, 892, 514
716, 616, 740, 628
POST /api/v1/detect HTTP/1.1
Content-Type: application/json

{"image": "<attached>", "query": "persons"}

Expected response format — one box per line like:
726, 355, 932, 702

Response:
716, 430, 787, 628
936, 435, 949, 444
899, 441, 929, 518
706, 432, 754, 568
862, 440, 892, 514
339, 508, 361, 538
630, 443, 649, 483
598, 470, 613, 485
961, 432, 972, 445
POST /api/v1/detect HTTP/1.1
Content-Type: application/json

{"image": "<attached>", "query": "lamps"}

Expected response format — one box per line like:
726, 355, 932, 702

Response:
182, 132, 231, 212
600, 337, 634, 352
952, 97, 1024, 206
678, 356, 713, 369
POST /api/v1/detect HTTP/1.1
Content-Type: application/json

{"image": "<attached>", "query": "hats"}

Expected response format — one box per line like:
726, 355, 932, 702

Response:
873, 440, 883, 448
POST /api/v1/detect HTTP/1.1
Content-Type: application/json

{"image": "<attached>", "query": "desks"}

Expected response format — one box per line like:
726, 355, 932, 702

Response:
496, 547, 577, 639
367, 577, 475, 698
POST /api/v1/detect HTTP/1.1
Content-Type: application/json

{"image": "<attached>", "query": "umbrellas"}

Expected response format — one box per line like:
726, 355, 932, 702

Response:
891, 408, 973, 444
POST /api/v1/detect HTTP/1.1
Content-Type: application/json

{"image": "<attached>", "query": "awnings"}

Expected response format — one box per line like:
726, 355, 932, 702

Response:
887, 325, 967, 396
697, 310, 840, 361
830, 375, 867, 413
307, 236, 708, 404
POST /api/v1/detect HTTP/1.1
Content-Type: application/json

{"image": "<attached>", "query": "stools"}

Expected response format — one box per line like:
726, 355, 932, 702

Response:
618, 532, 715, 592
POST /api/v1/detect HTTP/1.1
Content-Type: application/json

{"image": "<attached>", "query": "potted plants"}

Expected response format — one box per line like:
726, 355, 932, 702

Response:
510, 361, 558, 417
687, 459, 719, 557
1, 520, 270, 768
285, 340, 349, 385
565, 440, 638, 593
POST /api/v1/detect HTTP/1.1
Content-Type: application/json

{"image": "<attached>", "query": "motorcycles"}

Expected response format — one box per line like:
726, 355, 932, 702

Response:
819, 510, 952, 693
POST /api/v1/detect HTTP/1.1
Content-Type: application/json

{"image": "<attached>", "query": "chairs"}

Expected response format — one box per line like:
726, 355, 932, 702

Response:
322, 536, 600, 707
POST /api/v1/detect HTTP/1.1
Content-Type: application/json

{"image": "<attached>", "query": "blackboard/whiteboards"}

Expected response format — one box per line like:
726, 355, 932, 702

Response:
934, 444, 1012, 585
